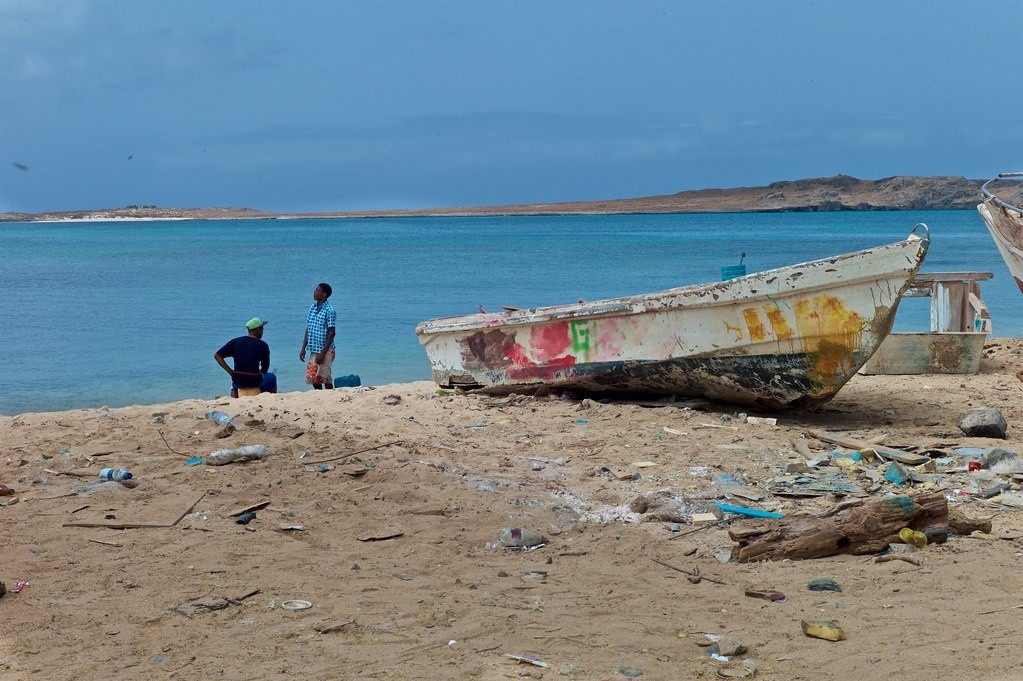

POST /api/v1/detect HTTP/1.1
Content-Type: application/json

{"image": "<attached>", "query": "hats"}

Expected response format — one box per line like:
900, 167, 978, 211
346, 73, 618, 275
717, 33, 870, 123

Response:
245, 317, 268, 330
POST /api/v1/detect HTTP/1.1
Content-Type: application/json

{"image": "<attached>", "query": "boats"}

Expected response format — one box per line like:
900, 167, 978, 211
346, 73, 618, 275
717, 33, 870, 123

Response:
415, 221, 932, 414
976, 171, 1023, 294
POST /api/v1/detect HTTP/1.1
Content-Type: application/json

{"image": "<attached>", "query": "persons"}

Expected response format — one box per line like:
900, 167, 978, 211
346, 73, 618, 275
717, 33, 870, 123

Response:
299, 283, 336, 390
214, 316, 277, 399
